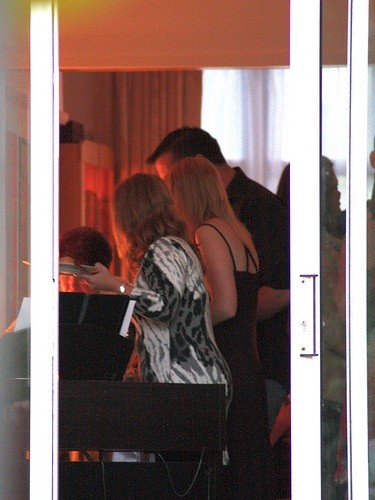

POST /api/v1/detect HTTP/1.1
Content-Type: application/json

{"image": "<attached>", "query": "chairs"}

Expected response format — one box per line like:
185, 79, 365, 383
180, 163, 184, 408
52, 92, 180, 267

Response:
57, 377, 228, 500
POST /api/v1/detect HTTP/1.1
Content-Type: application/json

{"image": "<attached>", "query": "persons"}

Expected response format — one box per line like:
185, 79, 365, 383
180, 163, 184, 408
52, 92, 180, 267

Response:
71, 172, 231, 467
161, 156, 274, 500
2, 227, 142, 462
274, 155, 346, 500
146, 127, 290, 427
337, 136, 374, 487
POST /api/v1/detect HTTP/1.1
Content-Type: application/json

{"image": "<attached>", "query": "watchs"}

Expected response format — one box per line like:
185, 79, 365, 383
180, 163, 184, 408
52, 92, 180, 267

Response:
115, 282, 126, 295
